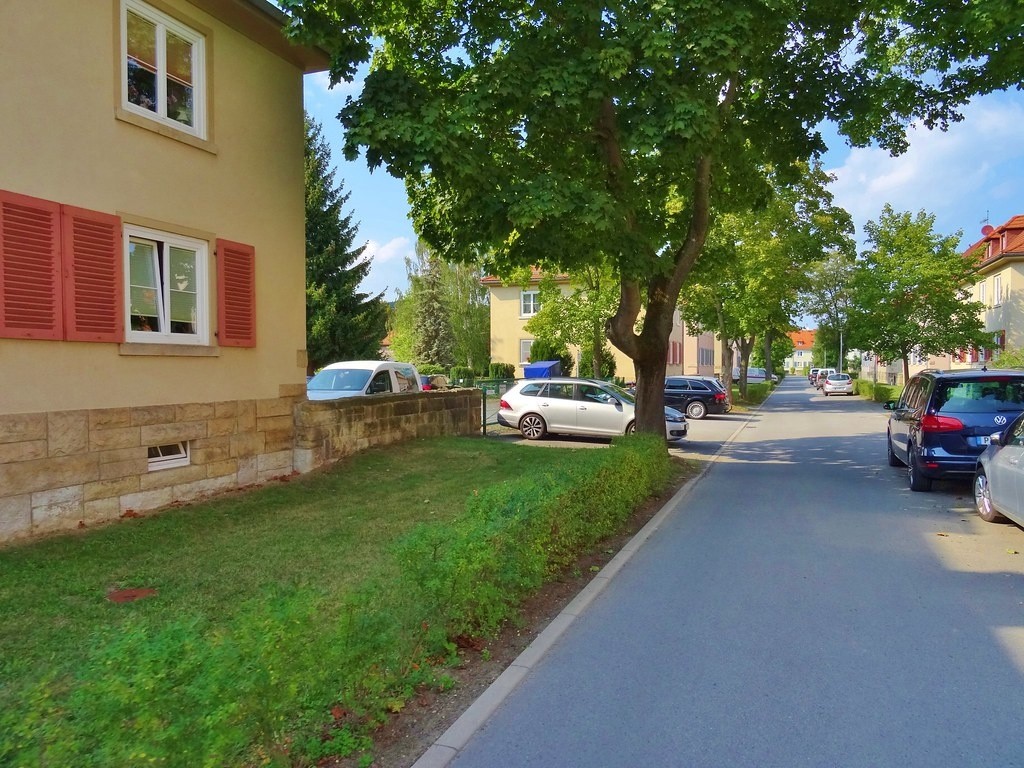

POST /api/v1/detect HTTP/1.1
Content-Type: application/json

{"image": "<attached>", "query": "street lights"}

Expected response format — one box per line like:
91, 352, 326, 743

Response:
839, 327, 844, 373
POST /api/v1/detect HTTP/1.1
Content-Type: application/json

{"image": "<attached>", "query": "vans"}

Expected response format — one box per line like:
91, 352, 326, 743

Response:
810, 368, 819, 384
815, 369, 836, 390
307, 360, 422, 400
732, 368, 778, 386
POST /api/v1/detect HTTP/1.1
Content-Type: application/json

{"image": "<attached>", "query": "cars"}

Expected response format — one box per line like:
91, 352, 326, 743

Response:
973, 410, 1024, 529
497, 377, 690, 441
419, 374, 461, 390
821, 374, 854, 396
599, 377, 733, 420
882, 368, 1024, 491
